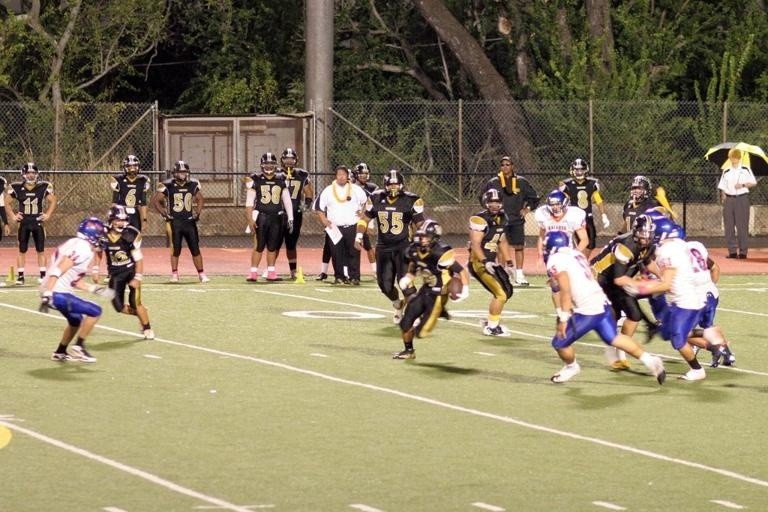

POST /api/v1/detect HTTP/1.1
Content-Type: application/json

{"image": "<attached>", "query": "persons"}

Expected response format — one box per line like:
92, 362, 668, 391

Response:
147, 159, 211, 285
108, 154, 152, 250
4, 161, 58, 285
268, 146, 314, 282
88, 203, 156, 341
242, 151, 294, 283
34, 216, 116, 364
715, 149, 758, 260
0, 174, 11, 242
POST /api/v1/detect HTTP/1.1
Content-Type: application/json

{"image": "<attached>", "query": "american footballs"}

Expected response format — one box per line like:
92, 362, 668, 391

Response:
447, 277, 463, 300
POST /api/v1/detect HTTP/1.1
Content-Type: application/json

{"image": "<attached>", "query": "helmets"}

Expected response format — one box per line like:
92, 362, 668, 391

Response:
542, 229, 570, 259
20, 161, 40, 184
76, 216, 107, 250
171, 160, 190, 183
122, 154, 140, 176
632, 209, 688, 243
630, 175, 654, 199
480, 188, 503, 213
349, 162, 372, 182
413, 218, 444, 248
385, 169, 403, 197
280, 148, 299, 169
570, 158, 589, 180
545, 189, 571, 218
107, 204, 130, 227
260, 153, 277, 176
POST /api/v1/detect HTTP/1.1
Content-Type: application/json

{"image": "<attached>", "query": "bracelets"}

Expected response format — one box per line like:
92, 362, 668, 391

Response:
134, 273, 144, 281
742, 184, 745, 188
305, 197, 312, 206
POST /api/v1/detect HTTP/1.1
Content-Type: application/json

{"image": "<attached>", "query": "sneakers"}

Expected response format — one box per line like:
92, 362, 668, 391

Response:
52, 305, 155, 363
515, 268, 530, 286
482, 318, 511, 337
650, 355, 667, 386
551, 362, 581, 383
678, 343, 737, 380
607, 349, 632, 370
393, 347, 415, 361
392, 297, 407, 324
439, 307, 450, 321
169, 271, 361, 285
16, 270, 25, 285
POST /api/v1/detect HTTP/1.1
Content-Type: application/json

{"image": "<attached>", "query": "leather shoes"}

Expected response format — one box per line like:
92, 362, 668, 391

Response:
726, 252, 748, 259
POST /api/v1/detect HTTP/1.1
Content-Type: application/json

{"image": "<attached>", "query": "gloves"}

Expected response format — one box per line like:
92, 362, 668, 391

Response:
484, 262, 498, 275
92, 285, 115, 301
288, 220, 294, 235
601, 213, 610, 229
448, 285, 469, 305
188, 212, 201, 223
161, 213, 174, 223
39, 291, 56, 312
504, 264, 517, 282
399, 275, 411, 291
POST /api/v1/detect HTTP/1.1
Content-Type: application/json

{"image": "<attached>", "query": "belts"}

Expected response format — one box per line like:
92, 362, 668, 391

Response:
725, 192, 748, 198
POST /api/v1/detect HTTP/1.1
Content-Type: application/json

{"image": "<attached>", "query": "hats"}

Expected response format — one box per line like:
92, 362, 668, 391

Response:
500, 156, 514, 167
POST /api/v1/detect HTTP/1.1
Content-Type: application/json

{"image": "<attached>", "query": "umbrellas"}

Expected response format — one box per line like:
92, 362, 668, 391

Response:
703, 141, 768, 177
